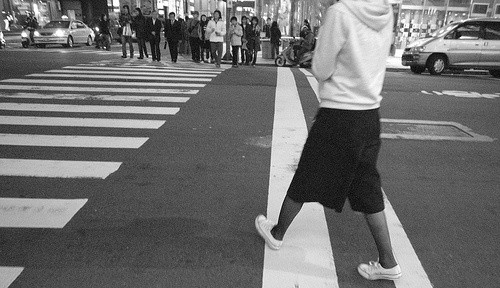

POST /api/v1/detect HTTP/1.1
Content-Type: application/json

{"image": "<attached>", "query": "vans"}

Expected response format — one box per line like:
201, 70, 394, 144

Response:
401, 18, 500, 75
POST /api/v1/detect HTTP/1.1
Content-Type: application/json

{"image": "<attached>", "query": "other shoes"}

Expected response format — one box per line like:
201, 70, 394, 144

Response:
255, 214, 285, 250
357, 256, 402, 281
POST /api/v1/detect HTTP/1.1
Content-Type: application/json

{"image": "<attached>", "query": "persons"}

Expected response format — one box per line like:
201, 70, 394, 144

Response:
176, 16, 194, 57
271, 21, 281, 60
254, 0, 403, 280
200, 15, 212, 64
293, 29, 314, 63
263, 17, 271, 39
187, 11, 200, 63
146, 10, 162, 62
239, 16, 251, 67
120, 5, 133, 58
0, 10, 319, 50
134, 7, 149, 59
164, 12, 182, 64
207, 9, 227, 69
249, 17, 261, 69
229, 16, 243, 67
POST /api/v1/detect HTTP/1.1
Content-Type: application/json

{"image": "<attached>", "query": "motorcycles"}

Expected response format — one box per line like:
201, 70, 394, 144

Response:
274, 36, 314, 67
93, 25, 113, 49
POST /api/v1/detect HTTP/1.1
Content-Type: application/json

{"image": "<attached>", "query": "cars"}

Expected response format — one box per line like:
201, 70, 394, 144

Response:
33, 15, 96, 48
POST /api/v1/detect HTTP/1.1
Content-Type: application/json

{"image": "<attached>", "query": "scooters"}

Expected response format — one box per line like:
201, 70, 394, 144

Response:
20, 25, 34, 48
